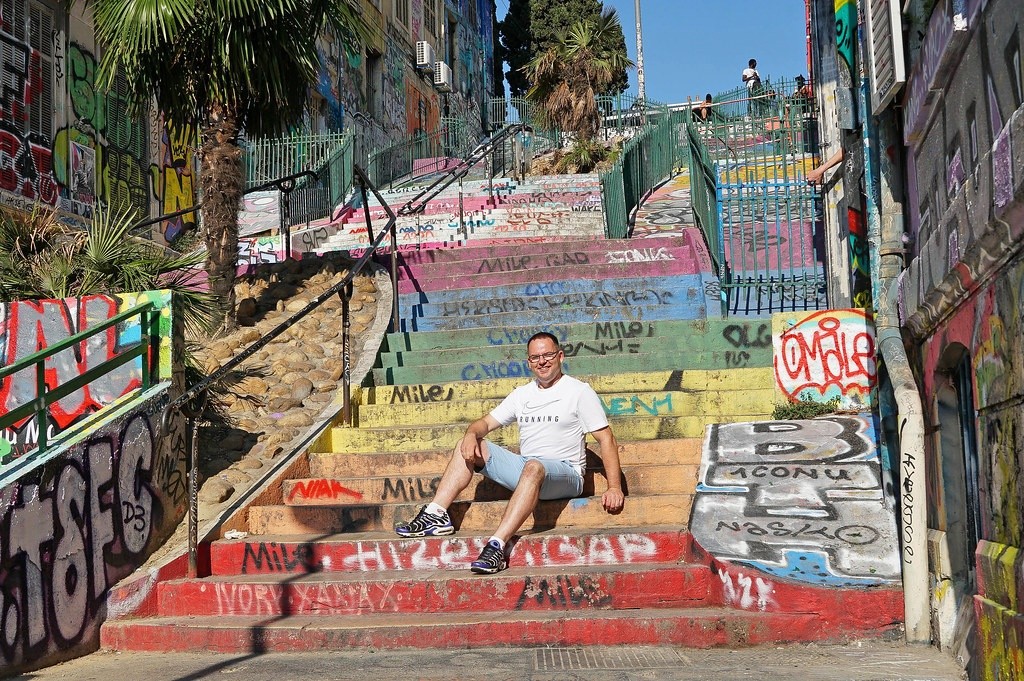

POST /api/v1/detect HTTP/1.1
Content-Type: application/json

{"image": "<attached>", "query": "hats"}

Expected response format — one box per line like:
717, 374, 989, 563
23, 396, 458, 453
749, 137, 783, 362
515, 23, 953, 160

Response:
706, 94, 711, 98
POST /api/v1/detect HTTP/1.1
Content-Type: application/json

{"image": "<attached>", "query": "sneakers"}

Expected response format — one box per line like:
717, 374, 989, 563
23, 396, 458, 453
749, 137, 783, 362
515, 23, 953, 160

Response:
470, 539, 507, 573
396, 504, 454, 537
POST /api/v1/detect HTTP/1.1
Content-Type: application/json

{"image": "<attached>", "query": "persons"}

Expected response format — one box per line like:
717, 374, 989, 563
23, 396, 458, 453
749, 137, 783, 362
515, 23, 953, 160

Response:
699, 94, 713, 122
791, 74, 809, 114
742, 59, 761, 116
396, 332, 625, 573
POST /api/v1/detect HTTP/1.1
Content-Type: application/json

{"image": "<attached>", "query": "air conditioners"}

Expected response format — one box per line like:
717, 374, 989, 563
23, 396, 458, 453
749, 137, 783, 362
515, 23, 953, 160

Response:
416, 41, 435, 73
434, 61, 453, 89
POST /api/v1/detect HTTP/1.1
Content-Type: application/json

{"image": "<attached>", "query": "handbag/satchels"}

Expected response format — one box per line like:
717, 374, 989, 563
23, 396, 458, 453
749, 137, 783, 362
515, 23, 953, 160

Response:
765, 90, 776, 99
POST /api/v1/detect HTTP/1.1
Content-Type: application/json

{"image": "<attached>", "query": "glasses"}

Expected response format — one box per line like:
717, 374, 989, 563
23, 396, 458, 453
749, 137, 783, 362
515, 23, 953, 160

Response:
528, 349, 559, 363
